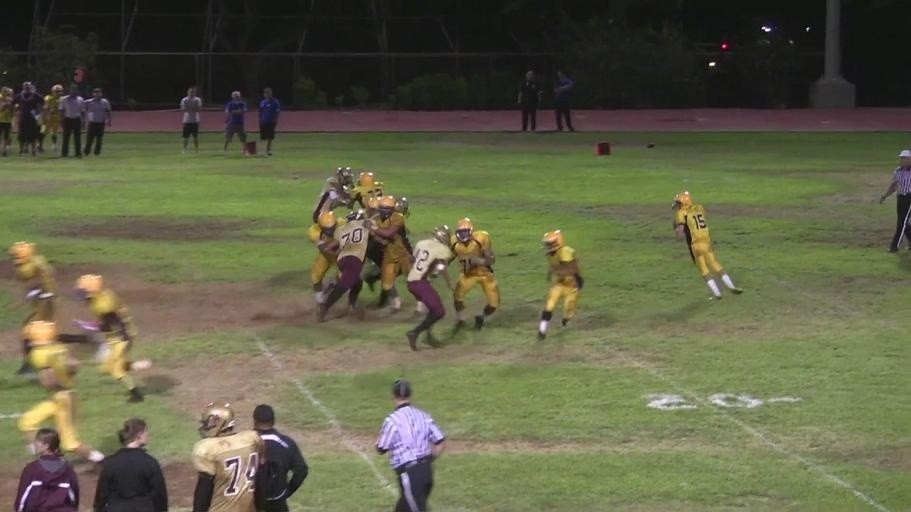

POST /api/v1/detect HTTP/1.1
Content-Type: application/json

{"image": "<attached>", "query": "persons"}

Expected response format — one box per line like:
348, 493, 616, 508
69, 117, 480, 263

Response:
376, 381, 446, 511
2, 81, 113, 155
179, 88, 202, 153
672, 191, 741, 299
259, 88, 280, 155
17, 320, 105, 469
222, 91, 247, 154
73, 274, 144, 403
93, 418, 168, 511
515, 71, 544, 131
552, 69, 576, 129
8, 240, 59, 374
13, 428, 80, 511
878, 150, 911, 252
308, 163, 500, 353
192, 402, 265, 511
536, 230, 582, 339
245, 405, 308, 511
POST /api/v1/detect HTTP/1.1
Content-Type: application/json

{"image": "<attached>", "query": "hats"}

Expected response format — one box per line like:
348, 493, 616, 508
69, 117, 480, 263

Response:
390, 376, 413, 399
897, 150, 911, 158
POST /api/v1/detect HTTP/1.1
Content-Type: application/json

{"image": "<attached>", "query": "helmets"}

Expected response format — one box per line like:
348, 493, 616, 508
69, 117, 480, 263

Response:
317, 210, 337, 237
432, 224, 451, 246
336, 166, 353, 185
51, 84, 64, 97
455, 217, 474, 245
542, 229, 564, 256
24, 320, 58, 347
672, 191, 692, 209
348, 209, 368, 221
7, 241, 35, 266
198, 400, 236, 439
71, 273, 104, 303
376, 195, 397, 219
359, 172, 376, 189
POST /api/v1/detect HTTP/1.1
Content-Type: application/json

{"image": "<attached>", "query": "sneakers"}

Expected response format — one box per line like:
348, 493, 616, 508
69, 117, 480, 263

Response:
730, 288, 744, 295
422, 336, 443, 349
90, 455, 108, 477
407, 330, 417, 351
453, 320, 464, 335
126, 394, 144, 404
538, 329, 547, 341
377, 291, 391, 308
317, 303, 328, 322
345, 303, 366, 320
132, 359, 150, 371
561, 320, 568, 326
474, 315, 483, 331
707, 295, 723, 302
362, 272, 378, 292
